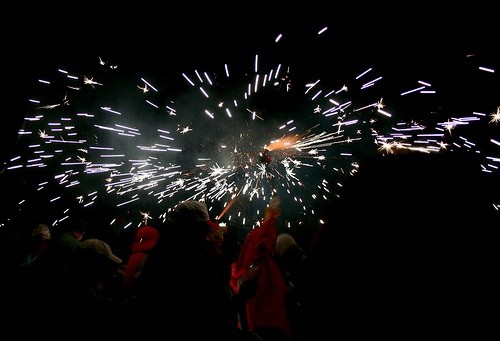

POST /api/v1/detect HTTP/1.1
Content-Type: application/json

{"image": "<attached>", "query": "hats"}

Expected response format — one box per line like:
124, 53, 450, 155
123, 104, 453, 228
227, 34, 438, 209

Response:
132, 226, 160, 251
67, 219, 86, 232
171, 201, 221, 231
77, 239, 122, 264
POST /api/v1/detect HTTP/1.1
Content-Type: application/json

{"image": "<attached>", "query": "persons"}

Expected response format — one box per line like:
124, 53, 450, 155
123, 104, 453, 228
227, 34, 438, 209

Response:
0, 195, 329, 341
